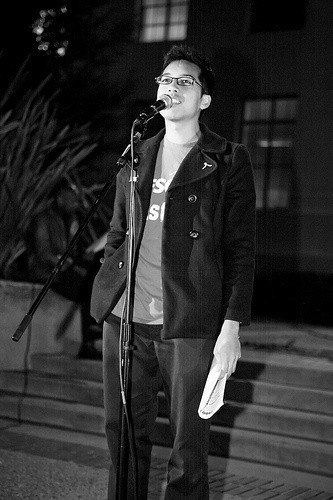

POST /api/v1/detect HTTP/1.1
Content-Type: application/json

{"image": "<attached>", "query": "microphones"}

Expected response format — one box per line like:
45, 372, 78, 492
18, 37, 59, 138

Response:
137, 94, 172, 124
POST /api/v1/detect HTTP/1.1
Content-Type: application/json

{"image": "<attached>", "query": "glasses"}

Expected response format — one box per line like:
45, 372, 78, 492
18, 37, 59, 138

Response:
155, 74, 206, 89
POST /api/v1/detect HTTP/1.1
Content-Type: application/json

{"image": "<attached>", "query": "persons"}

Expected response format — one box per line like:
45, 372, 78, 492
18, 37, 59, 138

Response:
89, 43, 258, 500
